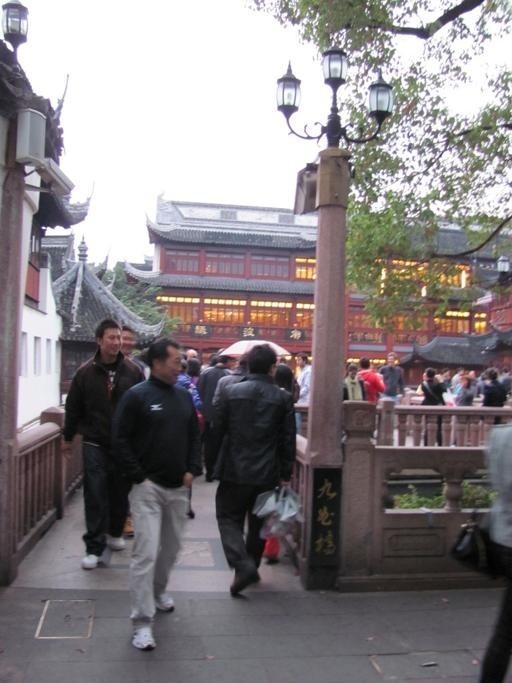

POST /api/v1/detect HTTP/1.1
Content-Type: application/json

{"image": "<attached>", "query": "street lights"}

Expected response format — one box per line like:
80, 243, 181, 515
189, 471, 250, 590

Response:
274, 39, 400, 469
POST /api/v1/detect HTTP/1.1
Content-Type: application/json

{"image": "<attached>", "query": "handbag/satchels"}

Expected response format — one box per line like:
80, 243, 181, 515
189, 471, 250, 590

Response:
451, 518, 503, 580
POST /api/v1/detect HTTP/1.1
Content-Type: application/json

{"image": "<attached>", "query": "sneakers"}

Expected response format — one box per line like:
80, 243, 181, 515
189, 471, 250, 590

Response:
155, 592, 175, 611
105, 534, 125, 550
132, 626, 157, 649
81, 554, 98, 569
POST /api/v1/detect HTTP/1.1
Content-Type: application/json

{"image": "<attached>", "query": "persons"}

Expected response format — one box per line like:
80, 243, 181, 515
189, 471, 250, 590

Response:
477, 418, 510, 683
197, 351, 507, 598
61, 318, 206, 652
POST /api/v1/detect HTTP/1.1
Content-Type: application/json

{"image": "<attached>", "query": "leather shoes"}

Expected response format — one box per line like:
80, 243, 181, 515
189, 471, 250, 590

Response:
122, 515, 134, 536
229, 566, 260, 595
